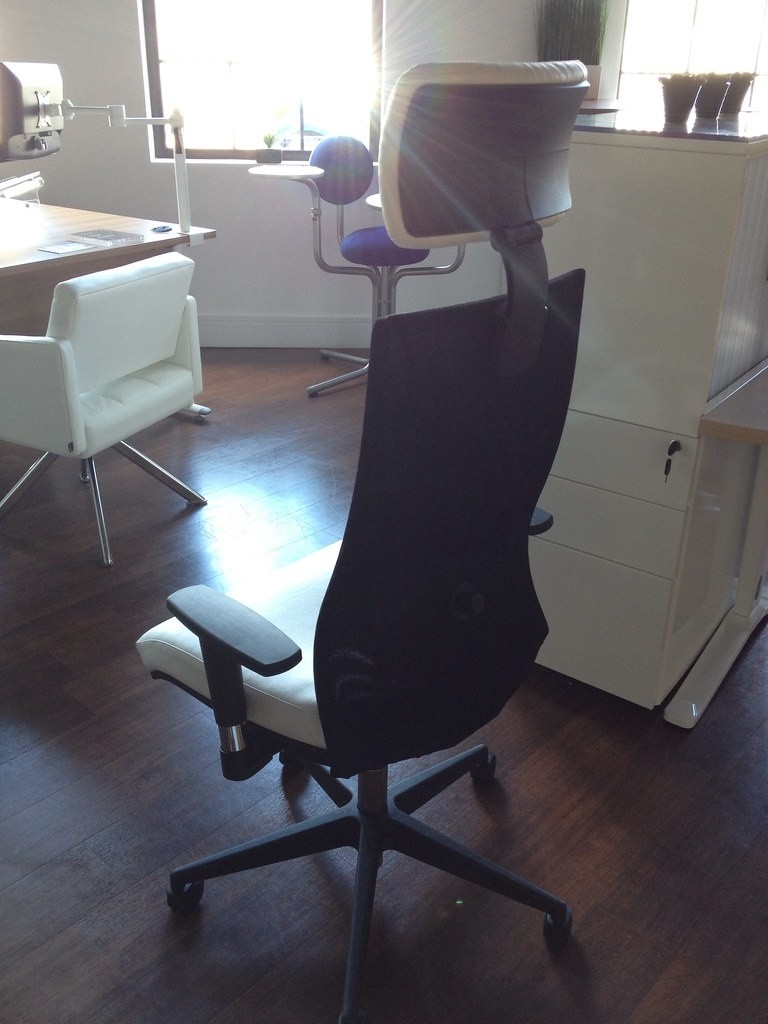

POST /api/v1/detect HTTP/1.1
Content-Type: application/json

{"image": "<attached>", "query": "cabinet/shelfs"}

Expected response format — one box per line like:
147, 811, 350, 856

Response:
498, 110, 768, 711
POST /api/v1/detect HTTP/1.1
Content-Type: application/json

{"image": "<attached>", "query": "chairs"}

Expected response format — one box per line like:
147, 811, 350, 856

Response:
0, 251, 207, 567
0, 196, 217, 426
248, 136, 466, 398
134, 58, 591, 1024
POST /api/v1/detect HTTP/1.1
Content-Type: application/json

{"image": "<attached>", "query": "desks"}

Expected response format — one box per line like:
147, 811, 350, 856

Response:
663, 355, 768, 730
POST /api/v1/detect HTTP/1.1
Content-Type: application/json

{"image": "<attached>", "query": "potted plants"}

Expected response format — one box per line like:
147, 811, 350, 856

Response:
660, 72, 759, 123
